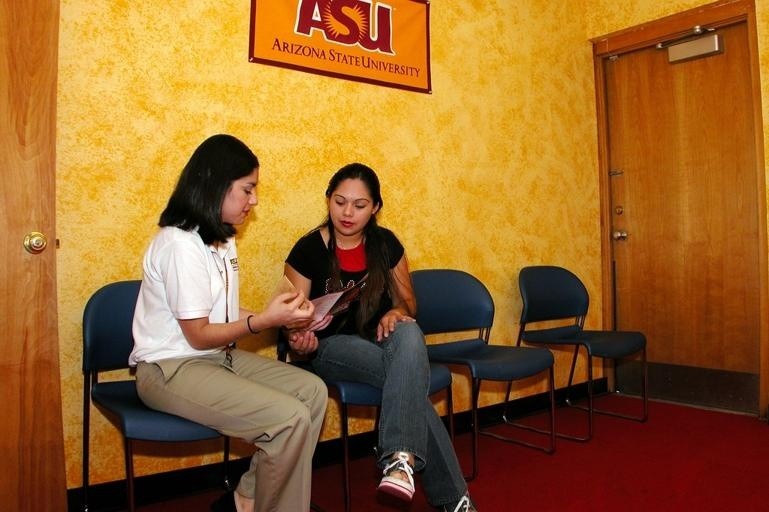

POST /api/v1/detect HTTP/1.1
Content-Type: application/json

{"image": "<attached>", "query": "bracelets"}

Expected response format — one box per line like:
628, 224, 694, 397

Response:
246, 315, 255, 335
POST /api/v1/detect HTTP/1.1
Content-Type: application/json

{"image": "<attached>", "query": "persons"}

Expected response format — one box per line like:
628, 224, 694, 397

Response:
284, 164, 474, 512
129, 135, 334, 511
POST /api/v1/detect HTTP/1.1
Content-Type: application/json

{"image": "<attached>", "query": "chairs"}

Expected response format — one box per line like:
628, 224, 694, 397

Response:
274, 289, 458, 511
410, 267, 557, 482
501, 266, 648, 444
80, 279, 232, 512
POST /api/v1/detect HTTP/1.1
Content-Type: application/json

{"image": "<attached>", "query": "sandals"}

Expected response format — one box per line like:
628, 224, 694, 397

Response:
376, 451, 415, 500
